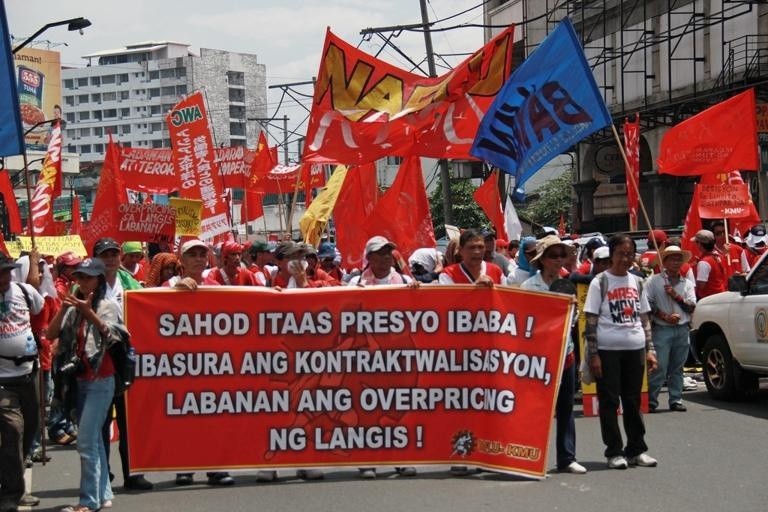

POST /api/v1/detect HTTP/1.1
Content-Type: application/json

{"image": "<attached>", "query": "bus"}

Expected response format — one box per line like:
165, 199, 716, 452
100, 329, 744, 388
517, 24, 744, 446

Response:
11, 194, 87, 230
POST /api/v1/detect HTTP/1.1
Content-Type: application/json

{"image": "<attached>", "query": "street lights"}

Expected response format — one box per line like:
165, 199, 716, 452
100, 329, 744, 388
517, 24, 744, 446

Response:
24, 117, 67, 137
14, 169, 40, 187
11, 16, 91, 56
10, 157, 45, 181
66, 163, 91, 225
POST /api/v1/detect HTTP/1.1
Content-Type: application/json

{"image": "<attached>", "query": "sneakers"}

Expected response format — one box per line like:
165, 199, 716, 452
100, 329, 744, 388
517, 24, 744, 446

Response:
449, 465, 467, 475
607, 455, 628, 469
174, 472, 235, 485
625, 451, 657, 467
18, 492, 41, 506
561, 461, 588, 474
22, 425, 79, 469
358, 466, 418, 479
123, 474, 154, 490
255, 470, 328, 482
669, 401, 687, 412
680, 375, 699, 392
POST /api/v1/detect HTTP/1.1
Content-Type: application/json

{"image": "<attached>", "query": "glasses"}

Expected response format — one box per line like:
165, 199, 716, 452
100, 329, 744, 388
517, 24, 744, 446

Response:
545, 250, 568, 261
613, 249, 635, 260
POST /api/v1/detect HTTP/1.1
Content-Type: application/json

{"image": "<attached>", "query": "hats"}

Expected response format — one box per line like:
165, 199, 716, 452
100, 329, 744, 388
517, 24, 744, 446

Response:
0, 250, 22, 274
688, 229, 716, 245
495, 238, 511, 248
750, 224, 767, 236
364, 236, 397, 256
55, 252, 81, 270
93, 236, 123, 258
219, 238, 345, 258
71, 257, 107, 280
483, 229, 497, 243
592, 246, 610, 261
536, 225, 559, 239
119, 240, 145, 256
647, 229, 672, 245
180, 238, 211, 257
657, 244, 692, 268
529, 234, 577, 267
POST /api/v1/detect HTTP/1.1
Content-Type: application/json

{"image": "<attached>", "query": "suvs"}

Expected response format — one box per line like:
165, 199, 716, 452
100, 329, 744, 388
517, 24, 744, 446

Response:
612, 228, 681, 271
689, 247, 768, 400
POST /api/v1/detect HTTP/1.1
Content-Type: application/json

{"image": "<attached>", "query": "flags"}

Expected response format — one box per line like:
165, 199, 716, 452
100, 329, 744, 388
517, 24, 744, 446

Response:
1, 1, 763, 271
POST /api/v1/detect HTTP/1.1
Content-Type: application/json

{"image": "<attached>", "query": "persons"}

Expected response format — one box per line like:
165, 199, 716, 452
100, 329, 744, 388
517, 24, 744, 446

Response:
1, 218, 766, 511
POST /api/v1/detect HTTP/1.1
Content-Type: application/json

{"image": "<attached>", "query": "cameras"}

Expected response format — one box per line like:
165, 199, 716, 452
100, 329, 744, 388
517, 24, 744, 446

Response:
57, 356, 85, 381
